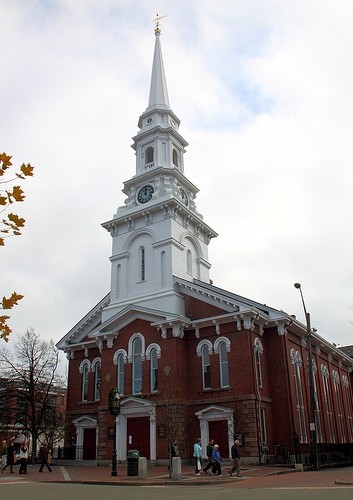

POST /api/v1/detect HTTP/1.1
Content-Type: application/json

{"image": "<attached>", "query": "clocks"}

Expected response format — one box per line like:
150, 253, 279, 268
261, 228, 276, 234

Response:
137, 185, 155, 204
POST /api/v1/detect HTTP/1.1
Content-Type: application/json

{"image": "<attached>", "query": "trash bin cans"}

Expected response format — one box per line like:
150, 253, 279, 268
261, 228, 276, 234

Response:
126, 450, 140, 476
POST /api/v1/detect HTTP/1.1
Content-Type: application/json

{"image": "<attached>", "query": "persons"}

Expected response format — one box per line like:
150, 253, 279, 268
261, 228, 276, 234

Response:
228, 440, 243, 477
192, 436, 203, 475
0, 439, 16, 473
168, 439, 180, 478
203, 439, 223, 476
19, 442, 29, 474
39, 444, 53, 473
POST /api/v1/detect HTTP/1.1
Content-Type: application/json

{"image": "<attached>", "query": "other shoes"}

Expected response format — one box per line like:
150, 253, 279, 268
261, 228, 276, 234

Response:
10, 471, 14, 474
203, 469, 208, 474
19, 472, 23, 474
217, 474, 223, 476
210, 470, 214, 476
195, 473, 201, 475
1, 469, 3, 474
39, 470, 43, 472
24, 472, 28, 474
237, 474, 243, 477
49, 469, 52, 472
229, 472, 233, 476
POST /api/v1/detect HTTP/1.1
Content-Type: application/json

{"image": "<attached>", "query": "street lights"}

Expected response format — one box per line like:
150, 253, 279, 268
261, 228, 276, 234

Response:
294, 282, 319, 471
108, 387, 122, 476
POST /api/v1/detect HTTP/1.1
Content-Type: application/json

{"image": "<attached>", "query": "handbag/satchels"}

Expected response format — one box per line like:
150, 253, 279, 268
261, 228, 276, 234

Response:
197, 459, 201, 470
